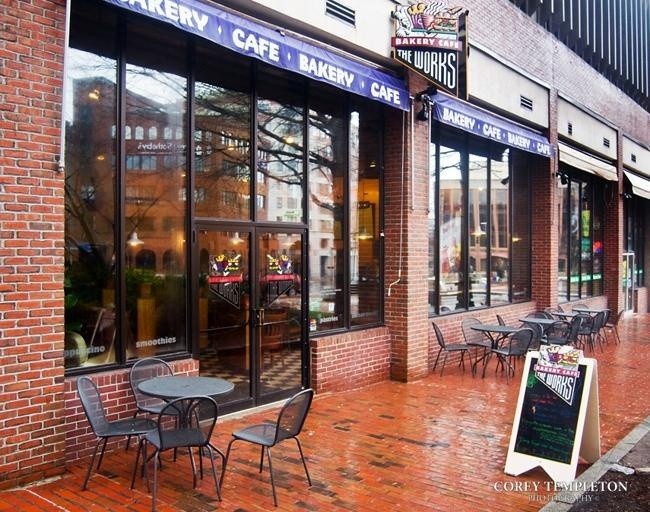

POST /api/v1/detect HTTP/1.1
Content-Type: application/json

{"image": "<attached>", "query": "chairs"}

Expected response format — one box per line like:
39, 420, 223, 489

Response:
524, 312, 549, 318
604, 310, 623, 345
518, 322, 542, 352
128, 358, 191, 463
485, 326, 533, 381
130, 395, 220, 506
569, 302, 590, 311
430, 322, 475, 377
571, 313, 596, 354
541, 320, 572, 345
74, 375, 158, 492
219, 386, 317, 506
460, 318, 490, 367
592, 312, 605, 353
497, 315, 518, 346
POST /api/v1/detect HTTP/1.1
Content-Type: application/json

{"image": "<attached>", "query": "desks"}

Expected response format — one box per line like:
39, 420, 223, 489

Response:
518, 317, 557, 329
138, 374, 238, 489
553, 312, 579, 322
571, 308, 605, 317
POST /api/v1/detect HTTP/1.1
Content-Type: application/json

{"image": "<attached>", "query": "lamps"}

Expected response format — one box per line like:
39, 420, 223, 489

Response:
354, 177, 373, 241
128, 191, 144, 247
472, 190, 485, 239
227, 228, 242, 245
